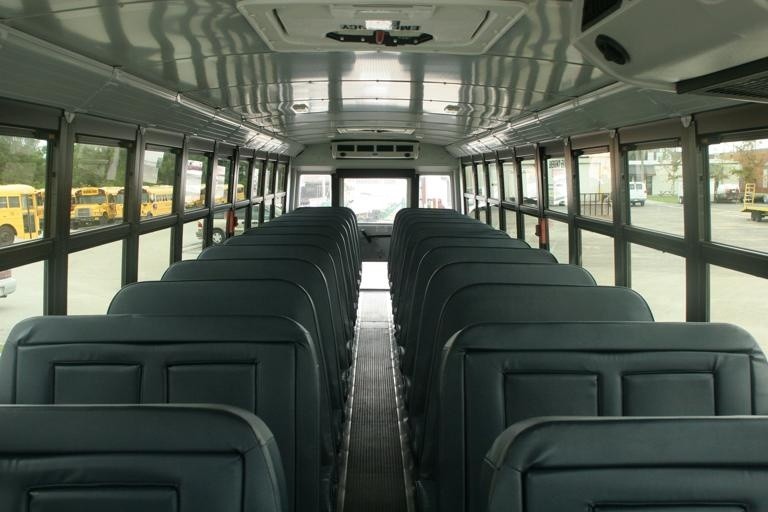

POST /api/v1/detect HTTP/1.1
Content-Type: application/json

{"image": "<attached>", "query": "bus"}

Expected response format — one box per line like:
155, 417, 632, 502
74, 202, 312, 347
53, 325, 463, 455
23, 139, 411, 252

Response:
1, 183, 247, 246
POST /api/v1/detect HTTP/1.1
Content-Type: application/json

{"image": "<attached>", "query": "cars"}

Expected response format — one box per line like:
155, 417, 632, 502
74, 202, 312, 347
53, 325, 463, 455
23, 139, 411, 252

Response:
195, 200, 282, 245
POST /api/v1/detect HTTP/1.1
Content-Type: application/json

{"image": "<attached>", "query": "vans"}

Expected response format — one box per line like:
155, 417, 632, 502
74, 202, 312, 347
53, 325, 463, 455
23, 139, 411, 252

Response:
629, 181, 647, 207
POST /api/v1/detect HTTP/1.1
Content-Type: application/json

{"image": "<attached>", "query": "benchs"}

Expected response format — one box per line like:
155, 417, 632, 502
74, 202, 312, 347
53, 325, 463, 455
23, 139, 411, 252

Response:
0, 208, 359, 512
388, 207, 768, 512
